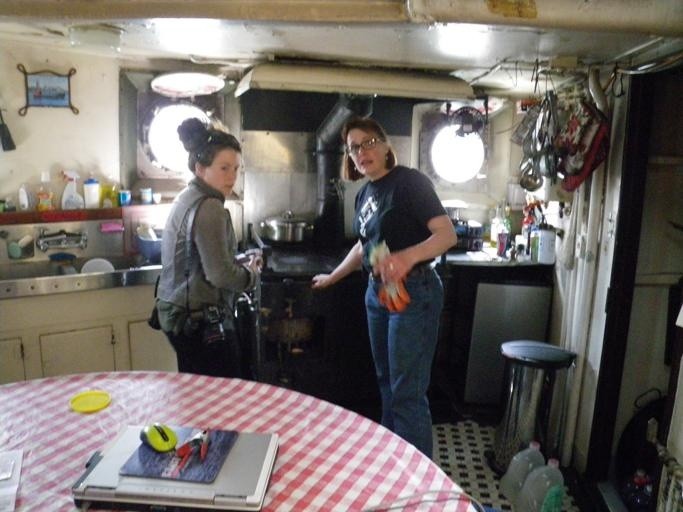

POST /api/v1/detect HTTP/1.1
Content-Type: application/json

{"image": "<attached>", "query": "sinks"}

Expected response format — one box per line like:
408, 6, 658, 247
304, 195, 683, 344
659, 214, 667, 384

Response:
69, 252, 163, 275
0, 259, 67, 282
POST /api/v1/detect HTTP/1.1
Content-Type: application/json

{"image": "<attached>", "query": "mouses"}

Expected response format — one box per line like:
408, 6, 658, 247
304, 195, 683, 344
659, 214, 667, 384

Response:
140, 422, 178, 453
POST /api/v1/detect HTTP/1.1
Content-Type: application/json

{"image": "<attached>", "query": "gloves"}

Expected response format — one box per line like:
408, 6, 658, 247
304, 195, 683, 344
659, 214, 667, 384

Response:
370, 242, 410, 311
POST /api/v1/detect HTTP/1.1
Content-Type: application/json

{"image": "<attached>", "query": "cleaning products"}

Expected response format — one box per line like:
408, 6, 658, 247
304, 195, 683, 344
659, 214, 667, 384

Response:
60, 170, 85, 208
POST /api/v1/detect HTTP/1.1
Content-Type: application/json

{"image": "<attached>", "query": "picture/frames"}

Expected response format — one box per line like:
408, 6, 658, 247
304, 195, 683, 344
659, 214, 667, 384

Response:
17, 63, 79, 115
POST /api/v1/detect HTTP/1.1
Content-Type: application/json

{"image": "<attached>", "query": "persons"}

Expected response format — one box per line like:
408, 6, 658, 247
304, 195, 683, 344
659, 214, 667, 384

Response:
153, 118, 265, 378
308, 117, 458, 461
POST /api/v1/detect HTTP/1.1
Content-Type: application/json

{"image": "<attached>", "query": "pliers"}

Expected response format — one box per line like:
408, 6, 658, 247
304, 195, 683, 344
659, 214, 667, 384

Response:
177, 427, 211, 461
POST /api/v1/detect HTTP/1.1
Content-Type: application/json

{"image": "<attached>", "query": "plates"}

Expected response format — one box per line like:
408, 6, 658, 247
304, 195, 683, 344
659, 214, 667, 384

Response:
80, 258, 115, 274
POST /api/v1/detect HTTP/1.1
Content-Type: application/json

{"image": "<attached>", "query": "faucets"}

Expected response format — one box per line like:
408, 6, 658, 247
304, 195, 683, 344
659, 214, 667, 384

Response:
33, 226, 88, 252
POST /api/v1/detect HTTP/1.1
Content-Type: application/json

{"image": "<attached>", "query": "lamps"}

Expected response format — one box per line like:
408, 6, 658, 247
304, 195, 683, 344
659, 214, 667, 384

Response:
68, 23, 127, 57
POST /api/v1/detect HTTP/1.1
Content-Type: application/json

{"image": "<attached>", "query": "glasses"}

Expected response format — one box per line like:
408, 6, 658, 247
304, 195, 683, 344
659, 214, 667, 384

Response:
345, 137, 383, 155
199, 133, 241, 150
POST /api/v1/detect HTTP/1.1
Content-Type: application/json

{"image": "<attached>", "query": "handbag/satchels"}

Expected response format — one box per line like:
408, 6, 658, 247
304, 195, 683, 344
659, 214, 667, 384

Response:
149, 307, 161, 333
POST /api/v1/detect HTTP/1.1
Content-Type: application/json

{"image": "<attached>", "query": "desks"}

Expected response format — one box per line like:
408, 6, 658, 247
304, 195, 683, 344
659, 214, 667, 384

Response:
0, 371, 478, 512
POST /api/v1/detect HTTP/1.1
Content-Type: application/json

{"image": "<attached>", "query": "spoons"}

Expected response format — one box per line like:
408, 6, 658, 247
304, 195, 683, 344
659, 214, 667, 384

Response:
259, 210, 316, 243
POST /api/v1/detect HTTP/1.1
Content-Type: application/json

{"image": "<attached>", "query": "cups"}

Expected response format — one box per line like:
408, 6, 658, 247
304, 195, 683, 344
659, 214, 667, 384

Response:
117, 190, 132, 207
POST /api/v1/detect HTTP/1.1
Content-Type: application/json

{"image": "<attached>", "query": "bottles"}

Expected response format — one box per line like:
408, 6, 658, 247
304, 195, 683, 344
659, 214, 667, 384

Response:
497, 228, 508, 257
491, 203, 513, 248
529, 226, 540, 263
17, 183, 34, 212
82, 178, 99, 209
536, 227, 557, 265
